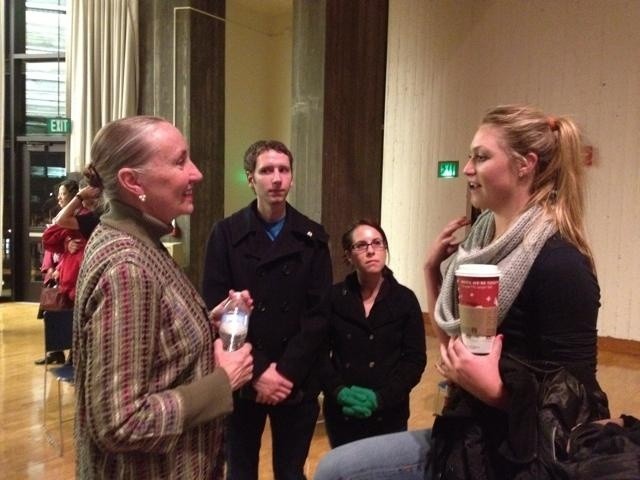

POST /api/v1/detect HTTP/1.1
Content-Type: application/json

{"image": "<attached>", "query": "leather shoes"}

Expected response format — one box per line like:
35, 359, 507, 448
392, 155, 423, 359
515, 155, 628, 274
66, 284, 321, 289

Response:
35, 351, 65, 365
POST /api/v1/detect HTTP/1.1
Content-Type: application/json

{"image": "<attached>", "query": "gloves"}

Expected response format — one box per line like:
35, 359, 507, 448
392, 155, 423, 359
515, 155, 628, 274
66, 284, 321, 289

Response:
332, 385, 378, 418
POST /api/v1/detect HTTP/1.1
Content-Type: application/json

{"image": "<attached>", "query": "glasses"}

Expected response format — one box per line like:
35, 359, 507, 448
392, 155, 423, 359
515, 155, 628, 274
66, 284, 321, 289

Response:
350, 237, 384, 251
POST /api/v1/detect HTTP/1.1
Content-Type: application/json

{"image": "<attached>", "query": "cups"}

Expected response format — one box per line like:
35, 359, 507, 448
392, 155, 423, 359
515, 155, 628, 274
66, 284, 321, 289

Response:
219, 308, 249, 352
454, 264, 502, 356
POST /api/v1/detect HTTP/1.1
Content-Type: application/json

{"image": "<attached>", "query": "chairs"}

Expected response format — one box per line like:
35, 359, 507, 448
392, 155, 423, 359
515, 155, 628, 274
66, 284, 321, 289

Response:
39, 309, 75, 457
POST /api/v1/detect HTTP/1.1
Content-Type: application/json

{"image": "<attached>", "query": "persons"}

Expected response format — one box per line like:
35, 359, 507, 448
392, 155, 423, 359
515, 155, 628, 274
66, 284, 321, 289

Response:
71, 114, 255, 479
206, 139, 332, 480
323, 219, 427, 449
312, 104, 610, 479
35, 178, 110, 381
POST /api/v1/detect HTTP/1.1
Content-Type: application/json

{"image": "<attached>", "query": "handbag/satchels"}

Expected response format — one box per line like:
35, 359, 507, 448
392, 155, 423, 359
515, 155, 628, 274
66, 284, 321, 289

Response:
40, 280, 65, 311
